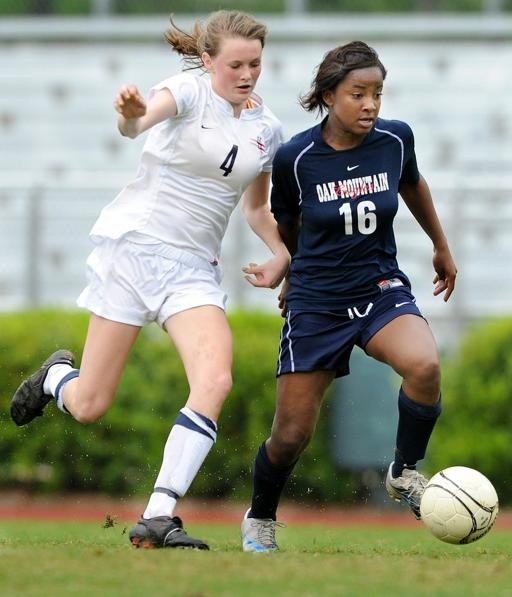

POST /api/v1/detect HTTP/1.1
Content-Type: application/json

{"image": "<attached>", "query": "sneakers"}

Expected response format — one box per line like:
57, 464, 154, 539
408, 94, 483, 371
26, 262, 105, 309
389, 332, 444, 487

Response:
384, 460, 429, 521
127, 513, 210, 550
11, 349, 75, 426
241, 506, 288, 554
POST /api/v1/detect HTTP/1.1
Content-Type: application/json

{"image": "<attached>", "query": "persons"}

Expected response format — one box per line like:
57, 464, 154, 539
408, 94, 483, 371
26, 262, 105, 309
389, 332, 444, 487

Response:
237, 37, 460, 553
5, 3, 294, 555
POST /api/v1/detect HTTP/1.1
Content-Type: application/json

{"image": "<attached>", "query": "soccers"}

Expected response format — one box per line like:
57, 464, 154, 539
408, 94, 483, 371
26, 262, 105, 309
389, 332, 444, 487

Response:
420, 465, 500, 545
